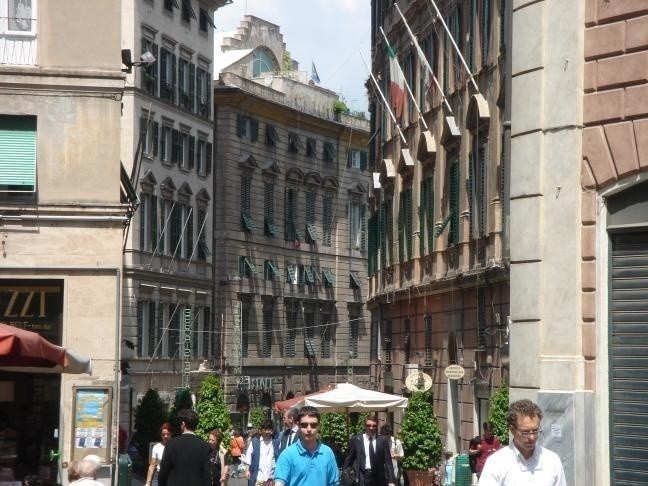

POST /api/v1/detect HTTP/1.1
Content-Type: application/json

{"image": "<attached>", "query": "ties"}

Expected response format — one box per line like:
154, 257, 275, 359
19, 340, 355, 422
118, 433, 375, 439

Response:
369, 436, 374, 465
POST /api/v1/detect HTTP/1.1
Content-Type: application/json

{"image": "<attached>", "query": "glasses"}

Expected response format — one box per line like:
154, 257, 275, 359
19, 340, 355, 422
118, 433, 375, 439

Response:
298, 422, 320, 429
514, 428, 540, 437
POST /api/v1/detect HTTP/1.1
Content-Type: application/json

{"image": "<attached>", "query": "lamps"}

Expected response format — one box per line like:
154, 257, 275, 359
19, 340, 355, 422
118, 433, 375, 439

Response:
129, 50, 157, 71
121, 339, 134, 351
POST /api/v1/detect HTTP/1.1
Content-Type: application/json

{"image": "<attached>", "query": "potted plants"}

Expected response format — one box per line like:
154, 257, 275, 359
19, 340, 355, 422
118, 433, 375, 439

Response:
393, 391, 443, 486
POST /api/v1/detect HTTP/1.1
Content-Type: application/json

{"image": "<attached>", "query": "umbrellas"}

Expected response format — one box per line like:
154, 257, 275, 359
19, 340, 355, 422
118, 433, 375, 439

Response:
295, 383, 408, 447
275, 389, 346, 440
0, 323, 90, 374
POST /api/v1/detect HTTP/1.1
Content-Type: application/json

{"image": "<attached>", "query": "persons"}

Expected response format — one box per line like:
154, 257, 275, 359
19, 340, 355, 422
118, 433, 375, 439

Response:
468, 421, 500, 486
476, 399, 566, 486
